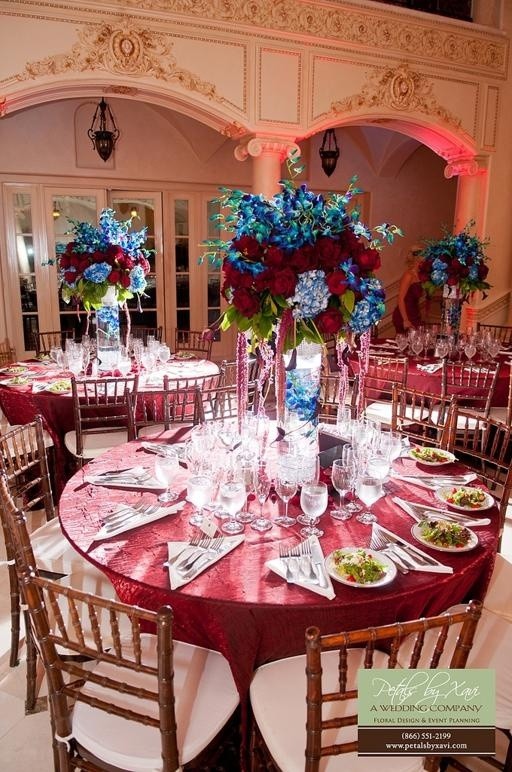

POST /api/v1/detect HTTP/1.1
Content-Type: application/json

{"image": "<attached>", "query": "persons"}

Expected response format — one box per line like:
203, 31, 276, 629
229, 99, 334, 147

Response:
394, 242, 430, 332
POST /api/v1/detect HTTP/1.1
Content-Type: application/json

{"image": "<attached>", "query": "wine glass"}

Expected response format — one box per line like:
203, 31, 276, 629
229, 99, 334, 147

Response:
332, 463, 356, 519
188, 419, 397, 490
187, 473, 211, 527
156, 453, 176, 503
301, 482, 326, 536
275, 469, 292, 525
357, 475, 378, 524
219, 478, 242, 531
254, 467, 271, 531
50, 332, 174, 374
392, 325, 503, 367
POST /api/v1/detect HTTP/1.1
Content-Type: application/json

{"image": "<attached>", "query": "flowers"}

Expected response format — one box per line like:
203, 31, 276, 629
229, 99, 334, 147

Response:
410, 215, 494, 305
41, 206, 153, 360
196, 148, 406, 436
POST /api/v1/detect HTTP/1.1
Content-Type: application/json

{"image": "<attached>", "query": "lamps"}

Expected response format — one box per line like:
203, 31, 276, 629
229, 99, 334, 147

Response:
88, 96, 121, 165
318, 128, 339, 178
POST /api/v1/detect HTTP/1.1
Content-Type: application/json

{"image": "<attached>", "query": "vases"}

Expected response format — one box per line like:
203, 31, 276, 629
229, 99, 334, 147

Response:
95, 284, 122, 370
438, 284, 463, 354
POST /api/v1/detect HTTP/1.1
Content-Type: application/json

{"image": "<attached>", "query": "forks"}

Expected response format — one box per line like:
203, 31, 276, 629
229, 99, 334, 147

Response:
97, 466, 153, 488
100, 499, 160, 529
164, 531, 225, 578
372, 530, 419, 577
276, 540, 318, 582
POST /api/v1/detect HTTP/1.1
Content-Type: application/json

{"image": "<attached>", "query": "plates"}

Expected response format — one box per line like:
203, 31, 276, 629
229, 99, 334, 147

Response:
413, 520, 480, 552
438, 485, 494, 511
328, 548, 398, 589
410, 446, 458, 466
1, 356, 72, 399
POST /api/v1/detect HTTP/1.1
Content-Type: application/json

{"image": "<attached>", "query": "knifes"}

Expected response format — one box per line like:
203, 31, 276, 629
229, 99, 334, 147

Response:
382, 529, 439, 568
